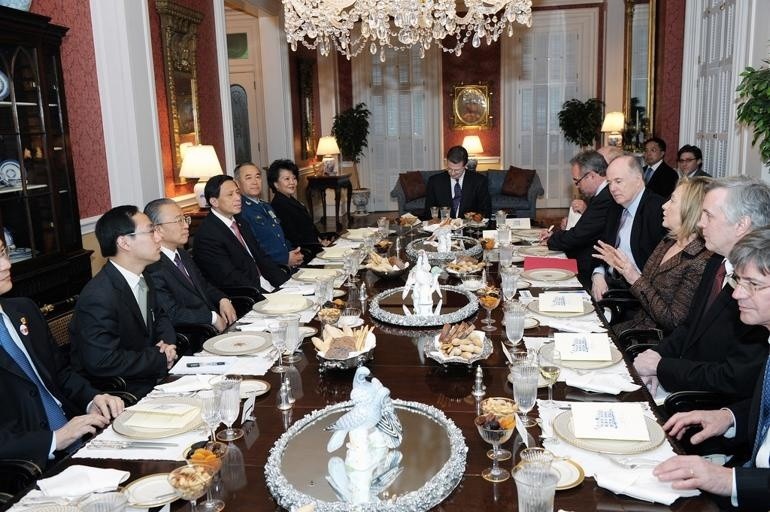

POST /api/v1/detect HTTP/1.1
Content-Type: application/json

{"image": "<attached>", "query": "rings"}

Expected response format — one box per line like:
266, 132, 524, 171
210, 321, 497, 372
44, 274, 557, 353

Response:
689, 468, 695, 478
620, 262, 625, 270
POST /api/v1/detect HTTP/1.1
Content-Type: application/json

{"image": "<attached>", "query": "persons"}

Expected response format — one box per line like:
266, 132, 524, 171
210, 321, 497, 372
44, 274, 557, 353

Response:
423, 145, 492, 219
641, 139, 679, 199
655, 230, 770, 511
144, 198, 243, 342
677, 144, 712, 178
560, 146, 624, 231
192, 174, 288, 309
70, 206, 178, 400
634, 175, 770, 408
588, 154, 669, 311
233, 163, 312, 277
539, 150, 622, 290
592, 176, 716, 347
269, 159, 340, 259
0, 234, 124, 471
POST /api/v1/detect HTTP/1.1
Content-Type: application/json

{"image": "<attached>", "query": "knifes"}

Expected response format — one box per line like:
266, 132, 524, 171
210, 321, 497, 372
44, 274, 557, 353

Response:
87, 445, 167, 450
90, 438, 179, 447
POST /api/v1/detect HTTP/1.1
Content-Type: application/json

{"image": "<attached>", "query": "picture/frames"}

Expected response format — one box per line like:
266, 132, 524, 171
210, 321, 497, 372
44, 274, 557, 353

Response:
448, 77, 495, 131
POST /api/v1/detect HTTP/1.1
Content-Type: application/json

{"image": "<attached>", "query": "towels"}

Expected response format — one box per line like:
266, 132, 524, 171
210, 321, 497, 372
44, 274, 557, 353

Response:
595, 461, 701, 505
566, 370, 642, 394
236, 318, 280, 333
37, 465, 130, 498
540, 317, 608, 333
154, 374, 226, 393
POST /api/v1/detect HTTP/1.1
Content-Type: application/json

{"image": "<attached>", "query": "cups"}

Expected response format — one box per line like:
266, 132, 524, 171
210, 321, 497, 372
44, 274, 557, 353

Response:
482, 241, 494, 267
500, 271, 518, 300
510, 350, 539, 412
276, 311, 303, 364
498, 230, 510, 246
377, 216, 391, 236
199, 389, 229, 457
343, 255, 356, 287
184, 441, 229, 512
536, 347, 563, 400
315, 275, 334, 307
440, 208, 451, 220
430, 206, 440, 219
479, 396, 517, 461
314, 279, 330, 321
77, 487, 130, 512
481, 296, 497, 332
481, 308, 496, 324
520, 446, 554, 470
502, 301, 527, 355
496, 216, 506, 228
466, 218, 475, 233
472, 219, 482, 235
510, 465, 561, 512
168, 463, 216, 511
500, 247, 513, 268
213, 373, 245, 441
268, 320, 291, 374
348, 253, 361, 283
474, 414, 516, 483
511, 367, 539, 428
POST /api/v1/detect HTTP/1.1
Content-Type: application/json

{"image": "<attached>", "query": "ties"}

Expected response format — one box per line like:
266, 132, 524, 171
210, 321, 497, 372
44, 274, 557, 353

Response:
0, 314, 82, 453
703, 260, 727, 321
174, 253, 193, 286
743, 353, 770, 467
452, 178, 462, 214
231, 221, 246, 249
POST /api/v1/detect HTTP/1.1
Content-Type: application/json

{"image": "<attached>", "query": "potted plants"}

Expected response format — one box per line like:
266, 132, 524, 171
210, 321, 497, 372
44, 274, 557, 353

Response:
558, 92, 607, 152
333, 101, 375, 217
737, 45, 770, 179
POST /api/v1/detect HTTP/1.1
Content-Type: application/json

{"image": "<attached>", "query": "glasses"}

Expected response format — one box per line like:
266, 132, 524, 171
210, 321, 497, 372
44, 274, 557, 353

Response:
725, 272, 770, 295
574, 169, 599, 186
117, 226, 158, 246
446, 167, 466, 173
1, 246, 13, 258
643, 148, 663, 152
679, 158, 697, 162
157, 216, 191, 227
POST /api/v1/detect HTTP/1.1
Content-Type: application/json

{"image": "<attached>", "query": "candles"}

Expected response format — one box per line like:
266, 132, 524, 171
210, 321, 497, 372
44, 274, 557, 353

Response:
305, 97, 313, 137
635, 109, 642, 145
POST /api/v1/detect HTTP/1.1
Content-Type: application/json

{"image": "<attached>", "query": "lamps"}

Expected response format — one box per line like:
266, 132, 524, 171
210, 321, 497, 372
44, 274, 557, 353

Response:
181, 145, 224, 207
601, 112, 627, 150
315, 137, 342, 174
282, 0, 533, 63
460, 136, 483, 162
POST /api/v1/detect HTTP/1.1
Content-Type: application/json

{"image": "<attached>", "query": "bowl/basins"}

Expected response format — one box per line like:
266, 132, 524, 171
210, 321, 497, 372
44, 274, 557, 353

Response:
340, 307, 362, 326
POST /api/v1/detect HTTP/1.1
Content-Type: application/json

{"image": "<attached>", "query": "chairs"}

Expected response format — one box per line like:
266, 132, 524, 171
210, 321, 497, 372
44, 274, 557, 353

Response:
305, 230, 344, 246
101, 331, 193, 388
218, 260, 287, 299
176, 294, 255, 341
283, 246, 318, 276
600, 289, 632, 303
0, 391, 140, 509
669, 396, 746, 451
627, 342, 711, 397
595, 298, 665, 342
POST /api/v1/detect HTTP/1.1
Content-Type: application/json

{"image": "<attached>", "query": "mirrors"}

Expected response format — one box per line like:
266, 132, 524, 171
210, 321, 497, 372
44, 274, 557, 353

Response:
622, 0, 655, 160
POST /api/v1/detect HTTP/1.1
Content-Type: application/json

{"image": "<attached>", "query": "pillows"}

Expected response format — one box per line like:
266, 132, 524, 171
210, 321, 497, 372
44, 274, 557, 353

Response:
398, 172, 427, 202
502, 166, 538, 198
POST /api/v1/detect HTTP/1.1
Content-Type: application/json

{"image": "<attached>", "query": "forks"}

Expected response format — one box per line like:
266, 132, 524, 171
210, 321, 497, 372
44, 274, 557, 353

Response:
21, 495, 82, 507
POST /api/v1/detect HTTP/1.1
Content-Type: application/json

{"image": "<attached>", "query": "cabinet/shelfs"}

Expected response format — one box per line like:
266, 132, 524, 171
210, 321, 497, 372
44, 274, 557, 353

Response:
0, 6, 92, 306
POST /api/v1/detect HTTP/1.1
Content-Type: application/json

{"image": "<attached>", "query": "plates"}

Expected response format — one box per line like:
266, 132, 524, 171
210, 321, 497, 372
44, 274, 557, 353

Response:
111, 403, 205, 439
511, 229, 544, 239
511, 459, 585, 492
0, 159, 28, 188
500, 315, 540, 330
239, 379, 272, 400
121, 472, 180, 509
518, 245, 564, 257
539, 341, 623, 371
0, 73, 12, 103
315, 250, 347, 262
333, 318, 365, 329
501, 279, 532, 290
552, 408, 666, 455
310, 348, 375, 374
423, 336, 495, 365
368, 261, 411, 281
519, 268, 576, 282
252, 294, 315, 316
202, 330, 273, 356
507, 372, 549, 388
445, 267, 484, 279
526, 299, 594, 318
290, 269, 337, 284
341, 232, 366, 242
389, 223, 411, 236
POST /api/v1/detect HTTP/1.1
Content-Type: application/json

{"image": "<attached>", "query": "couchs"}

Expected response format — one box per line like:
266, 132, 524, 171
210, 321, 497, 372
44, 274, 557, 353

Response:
390, 169, 545, 227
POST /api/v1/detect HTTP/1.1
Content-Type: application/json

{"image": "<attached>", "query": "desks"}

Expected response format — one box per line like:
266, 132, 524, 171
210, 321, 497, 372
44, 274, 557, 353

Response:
306, 174, 354, 225
